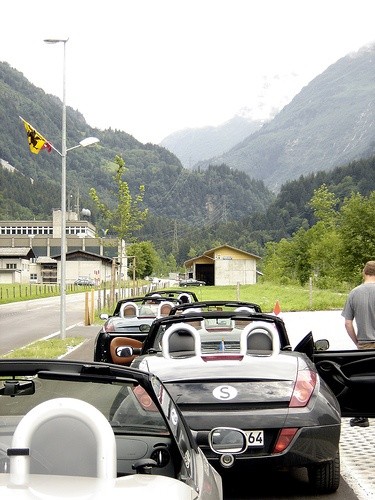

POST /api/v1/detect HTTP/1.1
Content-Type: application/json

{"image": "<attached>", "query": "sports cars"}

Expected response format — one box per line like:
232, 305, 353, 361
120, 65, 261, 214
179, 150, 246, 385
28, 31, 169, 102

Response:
0, 358, 223, 499
92, 288, 375, 494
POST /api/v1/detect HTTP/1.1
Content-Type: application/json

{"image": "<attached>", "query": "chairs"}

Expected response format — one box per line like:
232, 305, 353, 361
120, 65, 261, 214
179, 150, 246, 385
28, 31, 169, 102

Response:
177, 292, 192, 305
234, 306, 256, 314
240, 321, 280, 359
8, 395, 118, 500
120, 301, 140, 319
181, 307, 204, 314
161, 322, 202, 360
157, 301, 175, 317
151, 293, 163, 304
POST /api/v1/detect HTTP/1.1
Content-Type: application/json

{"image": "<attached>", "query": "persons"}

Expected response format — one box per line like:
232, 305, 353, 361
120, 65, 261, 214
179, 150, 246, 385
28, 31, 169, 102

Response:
341, 261, 375, 427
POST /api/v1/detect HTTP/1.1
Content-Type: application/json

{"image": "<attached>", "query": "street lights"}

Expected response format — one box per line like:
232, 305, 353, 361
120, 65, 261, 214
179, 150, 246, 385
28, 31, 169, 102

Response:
59, 137, 101, 340
43, 37, 68, 340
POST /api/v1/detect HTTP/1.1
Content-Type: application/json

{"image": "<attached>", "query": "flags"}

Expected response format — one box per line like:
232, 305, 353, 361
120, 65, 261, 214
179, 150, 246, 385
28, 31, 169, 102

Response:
24, 121, 51, 154
